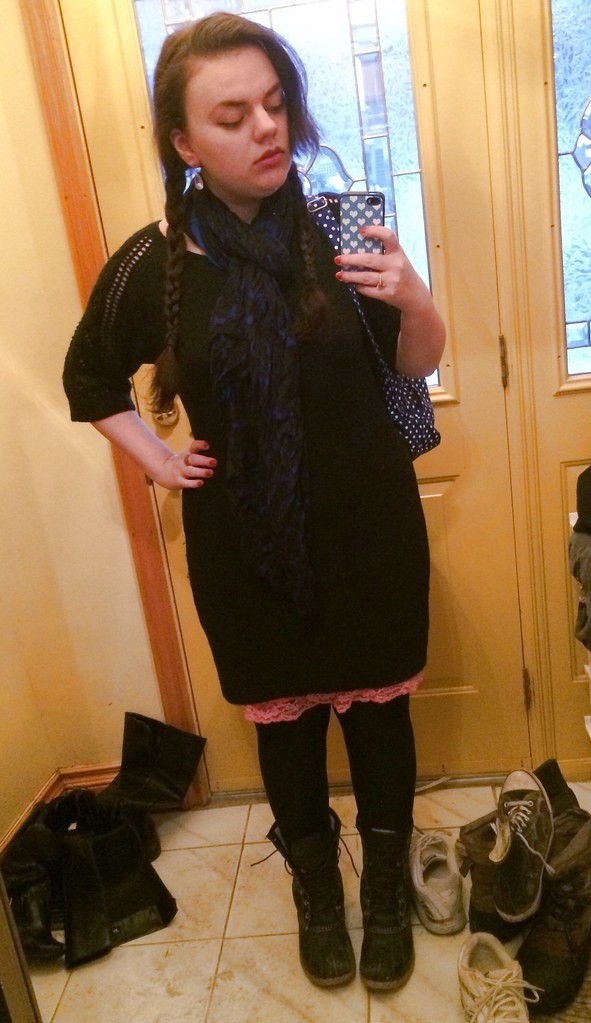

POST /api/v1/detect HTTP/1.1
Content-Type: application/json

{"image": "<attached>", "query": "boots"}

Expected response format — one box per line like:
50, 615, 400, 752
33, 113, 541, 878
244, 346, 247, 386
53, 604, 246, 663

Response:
263, 806, 357, 987
354, 809, 417, 991
455, 755, 580, 946
515, 808, 591, 1015
1, 711, 207, 970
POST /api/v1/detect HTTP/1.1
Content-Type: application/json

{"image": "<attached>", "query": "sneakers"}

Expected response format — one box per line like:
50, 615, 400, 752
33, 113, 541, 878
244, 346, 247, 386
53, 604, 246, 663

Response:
458, 930, 548, 1023
407, 825, 467, 935
488, 770, 554, 923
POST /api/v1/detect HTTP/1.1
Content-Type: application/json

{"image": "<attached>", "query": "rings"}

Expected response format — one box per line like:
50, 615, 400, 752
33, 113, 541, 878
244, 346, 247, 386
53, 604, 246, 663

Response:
184, 455, 191, 466
377, 274, 384, 289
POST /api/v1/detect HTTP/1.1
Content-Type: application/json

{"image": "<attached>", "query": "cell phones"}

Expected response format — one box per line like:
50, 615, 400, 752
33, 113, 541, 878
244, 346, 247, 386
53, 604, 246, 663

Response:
340, 191, 385, 272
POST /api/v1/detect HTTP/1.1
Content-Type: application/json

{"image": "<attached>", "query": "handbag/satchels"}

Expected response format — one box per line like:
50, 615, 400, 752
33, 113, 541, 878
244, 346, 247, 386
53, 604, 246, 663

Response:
382, 367, 441, 462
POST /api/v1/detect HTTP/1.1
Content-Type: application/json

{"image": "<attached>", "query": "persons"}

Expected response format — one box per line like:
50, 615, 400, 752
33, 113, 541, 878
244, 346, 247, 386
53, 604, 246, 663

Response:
61, 12, 447, 989
